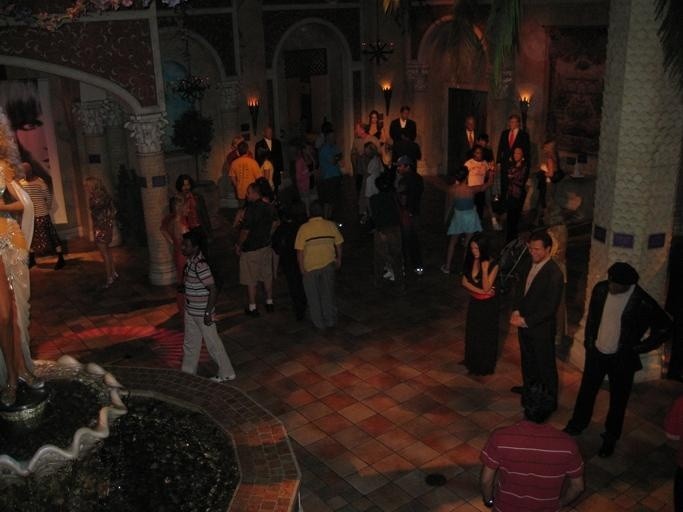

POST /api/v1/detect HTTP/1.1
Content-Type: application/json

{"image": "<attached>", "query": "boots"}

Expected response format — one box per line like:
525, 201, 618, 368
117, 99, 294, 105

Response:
53, 252, 66, 271
26, 252, 38, 270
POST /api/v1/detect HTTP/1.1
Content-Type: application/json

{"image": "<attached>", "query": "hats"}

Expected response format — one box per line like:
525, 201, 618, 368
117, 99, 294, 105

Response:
606, 261, 639, 285
508, 374, 559, 405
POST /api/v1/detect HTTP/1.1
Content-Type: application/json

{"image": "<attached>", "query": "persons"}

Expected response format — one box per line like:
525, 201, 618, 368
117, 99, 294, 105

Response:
477, 384, 583, 512
460, 229, 499, 376
361, 111, 385, 155
222, 135, 253, 174
561, 262, 670, 459
234, 183, 281, 316
359, 142, 424, 286
80, 175, 117, 288
294, 200, 344, 327
168, 175, 201, 293
507, 229, 563, 410
496, 148, 528, 243
496, 114, 531, 183
0, 154, 45, 409
178, 231, 237, 383
255, 121, 342, 233
440, 134, 495, 275
447, 116, 483, 180
18, 161, 64, 272
255, 127, 284, 171
390, 107, 417, 165
230, 142, 263, 208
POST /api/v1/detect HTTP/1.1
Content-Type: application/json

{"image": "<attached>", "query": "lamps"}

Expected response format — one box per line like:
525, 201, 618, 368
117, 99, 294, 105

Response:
380, 81, 397, 119
248, 98, 262, 138
516, 94, 529, 128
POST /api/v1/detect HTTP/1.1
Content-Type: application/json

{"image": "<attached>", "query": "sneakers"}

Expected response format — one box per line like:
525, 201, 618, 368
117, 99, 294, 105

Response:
103, 270, 120, 289
243, 304, 261, 319
208, 375, 235, 384
439, 263, 449, 275
263, 302, 275, 314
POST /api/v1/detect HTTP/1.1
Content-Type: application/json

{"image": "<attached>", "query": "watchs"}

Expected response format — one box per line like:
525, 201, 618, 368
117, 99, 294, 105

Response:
204, 312, 212, 317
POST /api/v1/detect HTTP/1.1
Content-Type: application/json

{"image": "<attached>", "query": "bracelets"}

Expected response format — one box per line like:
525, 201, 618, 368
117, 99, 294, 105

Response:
484, 498, 493, 505
